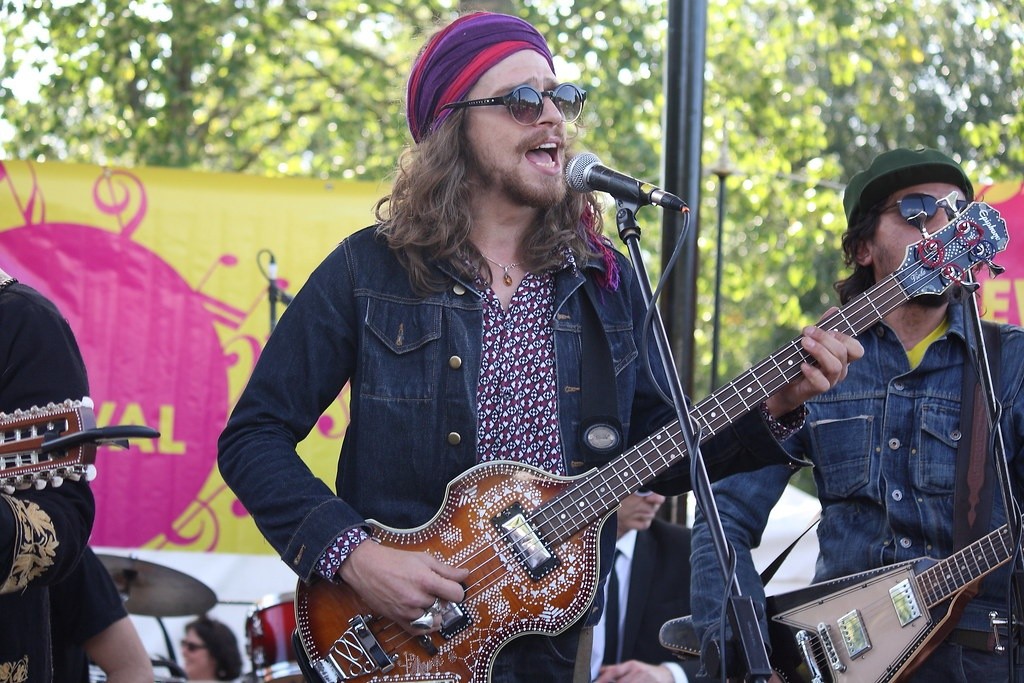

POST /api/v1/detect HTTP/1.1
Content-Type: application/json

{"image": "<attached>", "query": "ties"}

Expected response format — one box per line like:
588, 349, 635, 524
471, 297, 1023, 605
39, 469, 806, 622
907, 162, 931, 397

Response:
601, 549, 623, 666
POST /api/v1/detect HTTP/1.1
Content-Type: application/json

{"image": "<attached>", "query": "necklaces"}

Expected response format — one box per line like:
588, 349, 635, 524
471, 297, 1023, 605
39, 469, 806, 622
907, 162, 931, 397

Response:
482, 254, 526, 286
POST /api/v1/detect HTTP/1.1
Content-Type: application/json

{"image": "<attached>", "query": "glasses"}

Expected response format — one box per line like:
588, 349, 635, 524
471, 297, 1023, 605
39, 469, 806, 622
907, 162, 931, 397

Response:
435, 82, 589, 126
876, 193, 968, 221
180, 640, 207, 652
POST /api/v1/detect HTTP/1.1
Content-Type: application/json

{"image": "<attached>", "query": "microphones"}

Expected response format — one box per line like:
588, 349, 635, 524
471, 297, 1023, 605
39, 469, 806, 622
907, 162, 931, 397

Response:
565, 152, 690, 212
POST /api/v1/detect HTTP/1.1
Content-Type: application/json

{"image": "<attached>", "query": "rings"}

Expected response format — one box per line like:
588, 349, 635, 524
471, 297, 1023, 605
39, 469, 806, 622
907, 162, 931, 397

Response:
410, 612, 433, 629
425, 597, 442, 615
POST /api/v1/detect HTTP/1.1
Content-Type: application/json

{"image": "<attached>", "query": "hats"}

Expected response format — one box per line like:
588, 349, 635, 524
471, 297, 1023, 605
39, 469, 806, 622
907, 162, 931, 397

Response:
843, 146, 974, 229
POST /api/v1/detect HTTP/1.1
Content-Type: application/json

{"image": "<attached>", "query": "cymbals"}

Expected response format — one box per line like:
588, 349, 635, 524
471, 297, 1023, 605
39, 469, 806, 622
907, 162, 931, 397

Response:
95, 554, 216, 616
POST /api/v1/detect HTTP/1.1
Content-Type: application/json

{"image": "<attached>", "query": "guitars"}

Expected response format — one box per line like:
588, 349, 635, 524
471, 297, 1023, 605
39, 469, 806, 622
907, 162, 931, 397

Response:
659, 512, 1024, 683
293, 186, 1011, 683
0, 396, 160, 493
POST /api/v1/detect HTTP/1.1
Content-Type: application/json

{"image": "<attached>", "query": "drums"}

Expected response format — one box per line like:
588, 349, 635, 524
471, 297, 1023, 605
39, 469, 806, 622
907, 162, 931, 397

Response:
245, 591, 306, 683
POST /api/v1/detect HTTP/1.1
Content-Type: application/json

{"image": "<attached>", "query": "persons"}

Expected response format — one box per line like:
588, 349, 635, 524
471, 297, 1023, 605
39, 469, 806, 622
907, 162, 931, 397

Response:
217, 12, 862, 683
587, 490, 702, 683
180, 618, 244, 683
688, 149, 1024, 683
0, 266, 152, 683
